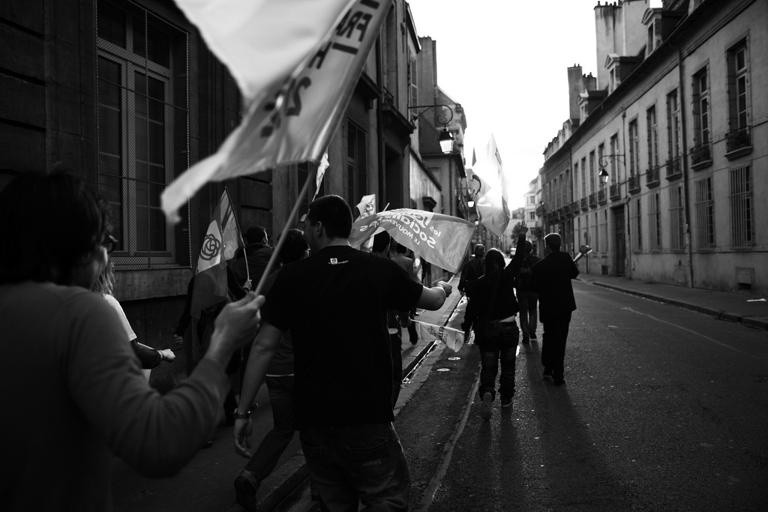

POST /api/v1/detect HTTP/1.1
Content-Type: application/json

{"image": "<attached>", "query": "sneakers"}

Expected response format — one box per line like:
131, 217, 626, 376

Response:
233, 471, 260, 511
523, 332, 565, 386
473, 387, 514, 409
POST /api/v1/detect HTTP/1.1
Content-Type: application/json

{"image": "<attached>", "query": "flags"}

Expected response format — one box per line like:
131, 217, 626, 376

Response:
471, 114, 510, 236
159, 0, 394, 228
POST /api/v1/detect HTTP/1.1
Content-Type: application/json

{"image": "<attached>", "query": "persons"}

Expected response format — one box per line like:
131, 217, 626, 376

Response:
174, 186, 435, 427
0, 162, 267, 511
231, 194, 455, 512
91, 258, 177, 370
456, 234, 581, 407
234, 227, 313, 512
